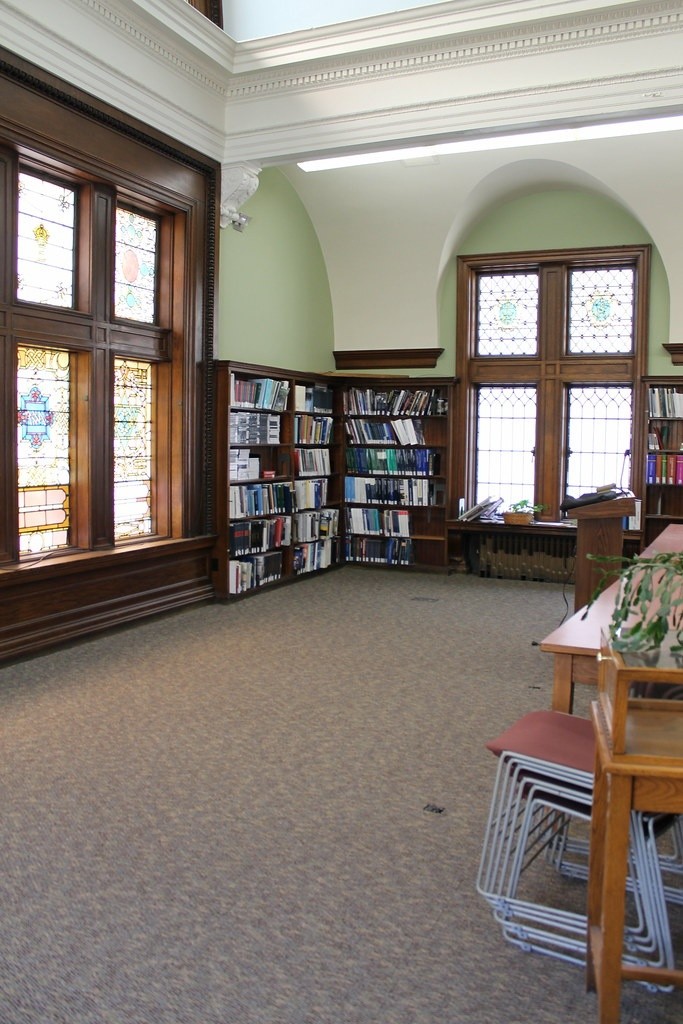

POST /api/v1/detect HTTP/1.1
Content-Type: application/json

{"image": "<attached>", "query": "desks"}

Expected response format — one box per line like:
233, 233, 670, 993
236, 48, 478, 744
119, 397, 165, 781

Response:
539, 522, 683, 848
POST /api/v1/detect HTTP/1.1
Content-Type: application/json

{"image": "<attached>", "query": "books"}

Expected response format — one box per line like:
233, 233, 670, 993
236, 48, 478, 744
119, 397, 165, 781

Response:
459, 496, 503, 521
622, 499, 642, 530
228, 372, 448, 594
648, 388, 683, 484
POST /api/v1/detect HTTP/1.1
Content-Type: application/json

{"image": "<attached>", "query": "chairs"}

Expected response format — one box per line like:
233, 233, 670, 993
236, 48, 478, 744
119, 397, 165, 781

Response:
475, 681, 683, 995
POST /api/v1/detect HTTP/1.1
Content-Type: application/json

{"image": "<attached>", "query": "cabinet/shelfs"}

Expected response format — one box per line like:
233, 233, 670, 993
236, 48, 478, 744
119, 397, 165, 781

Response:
216, 358, 334, 603
637, 373, 683, 552
334, 373, 462, 574
585, 624, 683, 1024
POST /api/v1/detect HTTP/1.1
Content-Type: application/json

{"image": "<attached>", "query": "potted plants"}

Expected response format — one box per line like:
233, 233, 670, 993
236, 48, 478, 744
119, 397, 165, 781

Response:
502, 499, 549, 526
